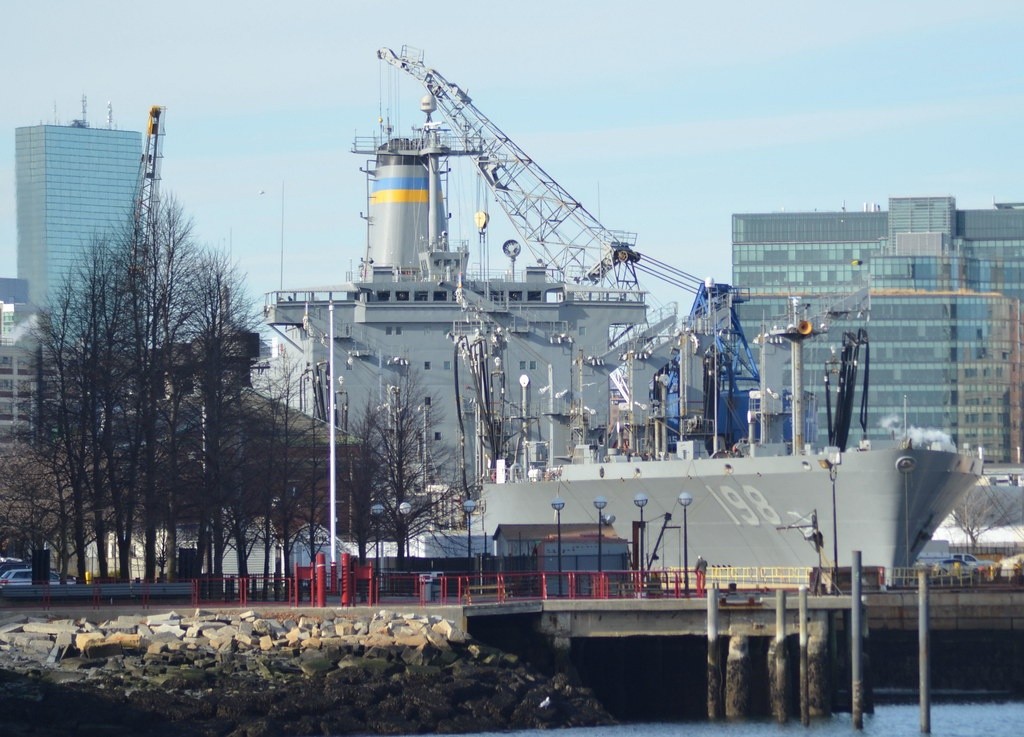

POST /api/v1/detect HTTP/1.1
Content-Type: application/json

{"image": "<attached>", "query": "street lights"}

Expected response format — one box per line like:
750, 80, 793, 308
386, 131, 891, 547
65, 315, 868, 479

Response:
399, 502, 412, 558
550, 497, 566, 597
633, 493, 649, 591
370, 504, 385, 571
818, 446, 842, 595
592, 495, 608, 572
463, 499, 475, 575
678, 492, 693, 598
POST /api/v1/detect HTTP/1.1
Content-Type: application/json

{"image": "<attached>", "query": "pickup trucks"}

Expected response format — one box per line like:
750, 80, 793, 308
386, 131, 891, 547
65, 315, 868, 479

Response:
950, 554, 979, 563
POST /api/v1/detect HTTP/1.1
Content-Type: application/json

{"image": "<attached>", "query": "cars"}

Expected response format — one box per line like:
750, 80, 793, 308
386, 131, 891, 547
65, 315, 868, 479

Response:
0, 557, 76, 585
939, 560, 979, 575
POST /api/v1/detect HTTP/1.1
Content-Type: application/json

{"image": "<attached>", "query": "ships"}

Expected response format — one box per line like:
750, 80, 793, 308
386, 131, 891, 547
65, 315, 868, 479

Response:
477, 277, 985, 582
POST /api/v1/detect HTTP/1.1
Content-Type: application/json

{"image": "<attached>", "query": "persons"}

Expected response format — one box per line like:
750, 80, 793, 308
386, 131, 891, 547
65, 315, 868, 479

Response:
694, 556, 707, 590
